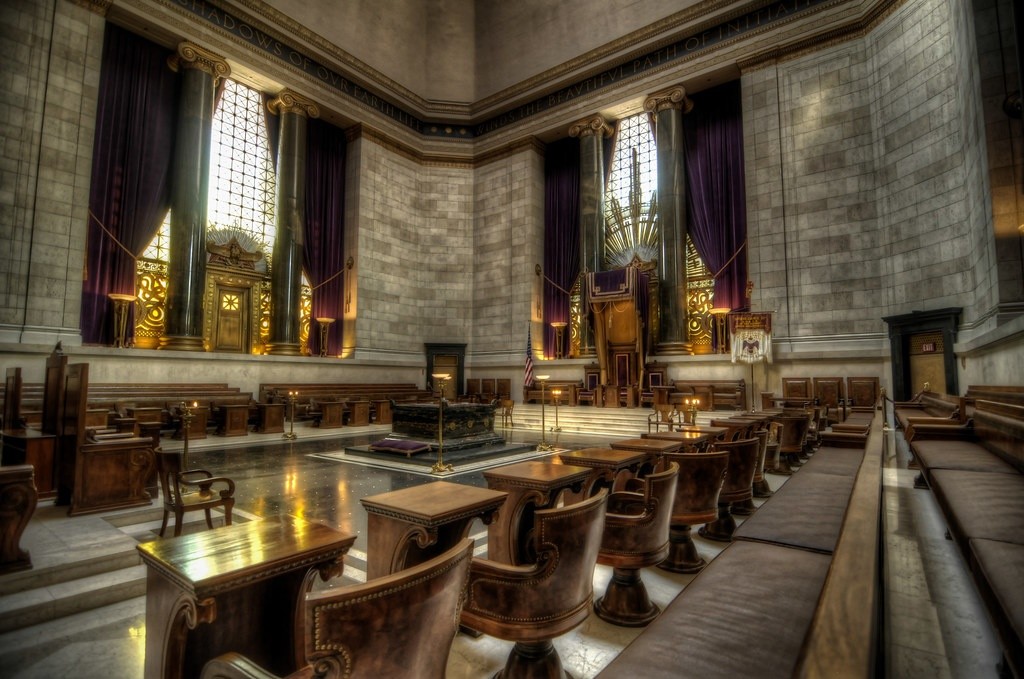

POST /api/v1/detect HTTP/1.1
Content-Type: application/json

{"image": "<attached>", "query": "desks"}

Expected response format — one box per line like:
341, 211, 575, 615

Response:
741, 414, 777, 425
675, 426, 729, 441
482, 461, 596, 566
136, 514, 357, 679
359, 481, 510, 582
710, 419, 757, 442
125, 408, 163, 437
345, 401, 369, 426
729, 416, 767, 431
172, 406, 208, 441
641, 432, 709, 448
215, 405, 252, 436
317, 402, 343, 428
253, 404, 286, 433
0, 428, 57, 498
559, 448, 651, 488
371, 400, 393, 424
610, 439, 683, 458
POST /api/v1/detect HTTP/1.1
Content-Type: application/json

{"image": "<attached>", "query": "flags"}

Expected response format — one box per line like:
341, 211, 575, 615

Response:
726, 313, 773, 365
523, 323, 534, 387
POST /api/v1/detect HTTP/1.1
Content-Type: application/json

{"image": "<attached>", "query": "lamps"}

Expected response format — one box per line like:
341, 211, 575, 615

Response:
316, 318, 336, 357
535, 375, 555, 452
428, 373, 455, 475
179, 402, 198, 469
108, 293, 137, 349
552, 386, 564, 432
551, 322, 567, 360
284, 391, 299, 440
708, 308, 731, 354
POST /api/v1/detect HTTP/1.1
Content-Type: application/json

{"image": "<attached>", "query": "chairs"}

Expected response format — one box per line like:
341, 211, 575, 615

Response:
764, 422, 784, 473
460, 488, 610, 679
154, 447, 235, 537
636, 451, 729, 575
648, 405, 697, 432
780, 377, 812, 408
782, 412, 822, 460
767, 417, 810, 476
603, 339, 638, 408
576, 362, 601, 407
3, 367, 28, 429
43, 341, 68, 431
200, 537, 474, 679
737, 428, 774, 498
54, 363, 153, 517
458, 378, 511, 407
594, 462, 680, 629
845, 377, 882, 418
695, 437, 760, 543
494, 400, 515, 428
641, 360, 668, 407
805, 377, 845, 423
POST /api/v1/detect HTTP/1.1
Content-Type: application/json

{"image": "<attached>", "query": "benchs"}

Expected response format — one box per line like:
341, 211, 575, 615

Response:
894, 392, 967, 451
668, 378, 747, 411
523, 378, 584, 407
0, 383, 256, 418
831, 394, 886, 433
258, 383, 434, 421
593, 411, 885, 679
907, 400, 1024, 679
